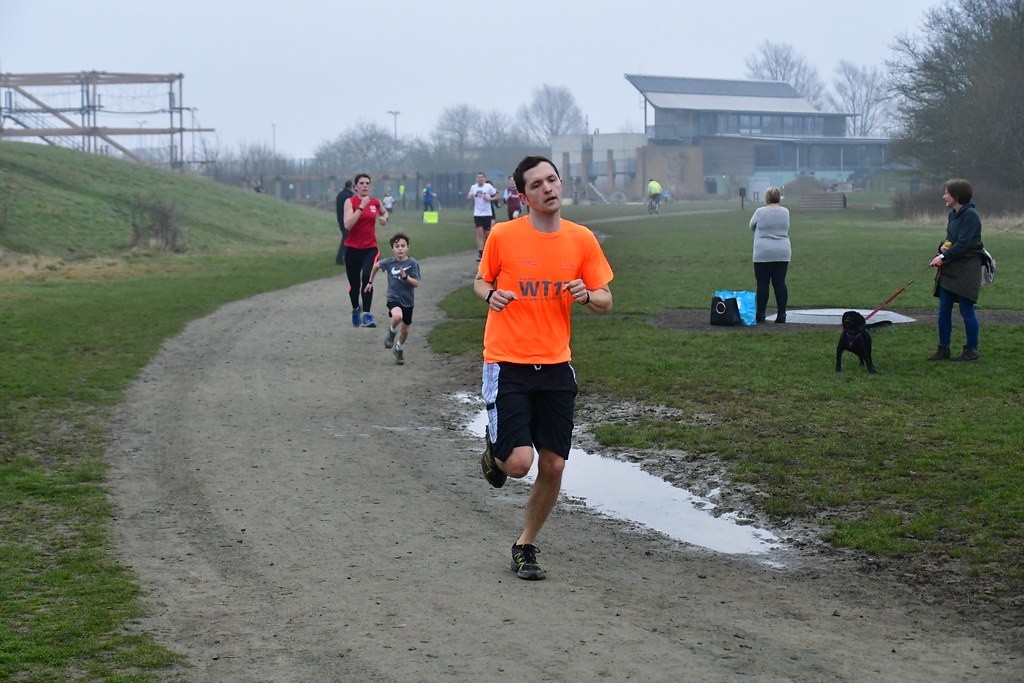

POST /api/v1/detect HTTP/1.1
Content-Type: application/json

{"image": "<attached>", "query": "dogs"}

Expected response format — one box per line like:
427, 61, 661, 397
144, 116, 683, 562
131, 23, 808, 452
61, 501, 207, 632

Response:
835, 311, 876, 374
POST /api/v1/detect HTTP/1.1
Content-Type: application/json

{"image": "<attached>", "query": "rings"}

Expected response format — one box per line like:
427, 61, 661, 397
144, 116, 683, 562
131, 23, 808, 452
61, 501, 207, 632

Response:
576, 293, 579, 298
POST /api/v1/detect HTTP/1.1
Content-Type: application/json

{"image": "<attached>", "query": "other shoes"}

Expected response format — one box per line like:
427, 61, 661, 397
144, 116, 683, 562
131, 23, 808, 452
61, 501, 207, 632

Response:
775, 315, 786, 323
756, 313, 766, 323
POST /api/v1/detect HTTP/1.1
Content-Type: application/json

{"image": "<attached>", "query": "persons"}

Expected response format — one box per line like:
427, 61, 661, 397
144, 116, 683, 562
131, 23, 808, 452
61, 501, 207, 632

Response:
925, 179, 984, 360
344, 174, 389, 328
423, 183, 437, 211
381, 180, 407, 213
662, 187, 671, 207
467, 172, 501, 262
503, 175, 522, 220
364, 233, 421, 365
647, 178, 662, 212
474, 155, 612, 580
749, 186, 792, 324
335, 180, 354, 264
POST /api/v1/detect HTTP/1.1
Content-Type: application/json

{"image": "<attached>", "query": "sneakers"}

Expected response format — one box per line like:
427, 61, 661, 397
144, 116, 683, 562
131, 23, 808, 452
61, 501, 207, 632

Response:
956, 345, 980, 361
476, 254, 482, 261
927, 345, 950, 361
385, 329, 396, 349
481, 426, 507, 488
352, 307, 361, 327
511, 543, 545, 580
394, 345, 405, 364
362, 314, 376, 328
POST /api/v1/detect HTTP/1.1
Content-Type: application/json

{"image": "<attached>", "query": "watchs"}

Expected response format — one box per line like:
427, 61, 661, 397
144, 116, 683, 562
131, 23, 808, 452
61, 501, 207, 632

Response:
940, 254, 945, 262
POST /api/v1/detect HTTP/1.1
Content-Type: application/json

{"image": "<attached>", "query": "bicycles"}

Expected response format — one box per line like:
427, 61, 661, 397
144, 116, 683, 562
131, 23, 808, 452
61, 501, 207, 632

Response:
648, 196, 661, 214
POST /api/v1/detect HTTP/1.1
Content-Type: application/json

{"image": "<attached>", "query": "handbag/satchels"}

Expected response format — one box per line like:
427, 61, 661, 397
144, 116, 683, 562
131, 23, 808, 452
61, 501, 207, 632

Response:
714, 291, 757, 327
709, 296, 744, 326
981, 247, 996, 287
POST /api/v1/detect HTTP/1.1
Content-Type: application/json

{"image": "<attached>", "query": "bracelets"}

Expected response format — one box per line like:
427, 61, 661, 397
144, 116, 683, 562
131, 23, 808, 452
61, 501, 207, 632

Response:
403, 275, 407, 280
385, 217, 389, 223
367, 281, 372, 284
578, 292, 589, 305
484, 290, 497, 304
357, 206, 363, 211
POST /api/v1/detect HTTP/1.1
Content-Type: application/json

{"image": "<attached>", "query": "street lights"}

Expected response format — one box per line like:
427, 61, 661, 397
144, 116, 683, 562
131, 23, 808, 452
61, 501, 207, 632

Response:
387, 110, 400, 140
